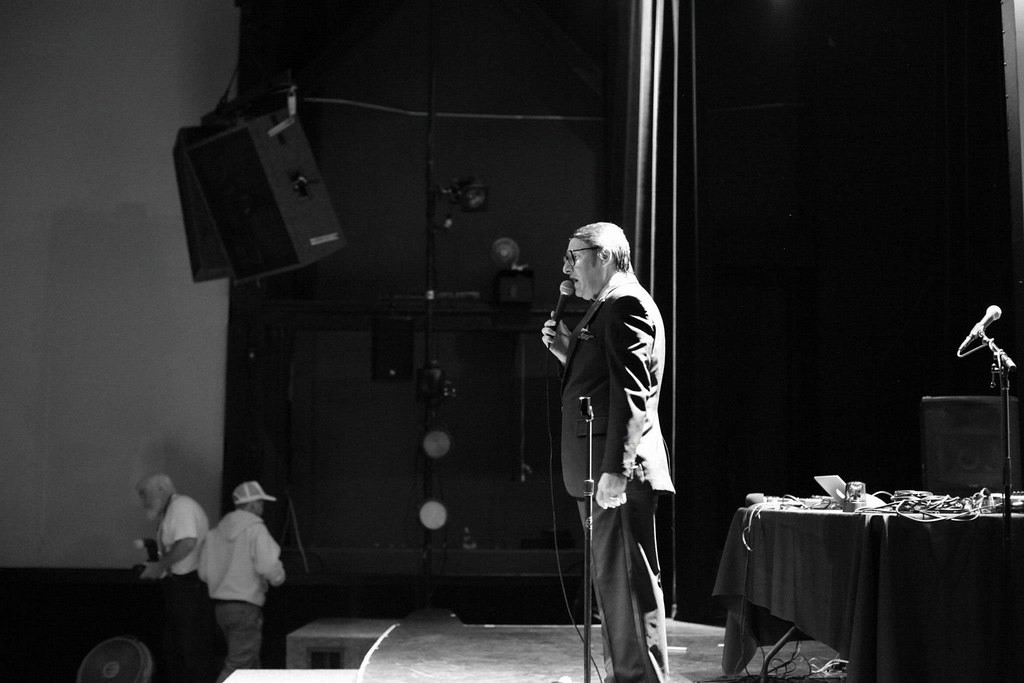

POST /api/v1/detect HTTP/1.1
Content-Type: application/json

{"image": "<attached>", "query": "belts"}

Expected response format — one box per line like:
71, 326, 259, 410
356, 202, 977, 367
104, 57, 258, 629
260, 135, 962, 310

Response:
215, 598, 247, 604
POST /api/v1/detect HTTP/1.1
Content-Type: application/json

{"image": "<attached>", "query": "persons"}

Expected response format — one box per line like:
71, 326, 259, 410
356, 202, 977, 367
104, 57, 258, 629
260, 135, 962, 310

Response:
196, 482, 285, 682
541, 219, 677, 683
135, 473, 217, 683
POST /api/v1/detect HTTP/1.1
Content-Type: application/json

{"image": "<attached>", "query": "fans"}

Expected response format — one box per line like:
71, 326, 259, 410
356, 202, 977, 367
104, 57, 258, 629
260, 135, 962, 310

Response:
77, 632, 153, 683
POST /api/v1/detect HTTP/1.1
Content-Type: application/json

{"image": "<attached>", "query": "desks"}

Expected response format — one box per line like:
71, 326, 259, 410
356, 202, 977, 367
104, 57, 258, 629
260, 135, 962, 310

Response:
726, 508, 1024, 683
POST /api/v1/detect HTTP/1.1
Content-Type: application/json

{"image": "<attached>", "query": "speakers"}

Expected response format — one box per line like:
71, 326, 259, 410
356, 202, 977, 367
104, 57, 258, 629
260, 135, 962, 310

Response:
186, 102, 340, 285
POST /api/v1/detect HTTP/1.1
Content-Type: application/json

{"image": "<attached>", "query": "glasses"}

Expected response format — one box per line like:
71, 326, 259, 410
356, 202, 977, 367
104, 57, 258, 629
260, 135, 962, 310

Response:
563, 246, 601, 266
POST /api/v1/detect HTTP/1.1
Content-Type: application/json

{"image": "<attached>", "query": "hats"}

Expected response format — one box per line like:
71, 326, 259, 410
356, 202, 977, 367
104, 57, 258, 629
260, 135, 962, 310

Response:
232, 480, 276, 504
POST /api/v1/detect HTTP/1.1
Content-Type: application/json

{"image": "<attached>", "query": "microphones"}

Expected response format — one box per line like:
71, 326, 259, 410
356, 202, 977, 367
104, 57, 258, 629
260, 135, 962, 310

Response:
548, 280, 574, 342
956, 304, 1002, 353
